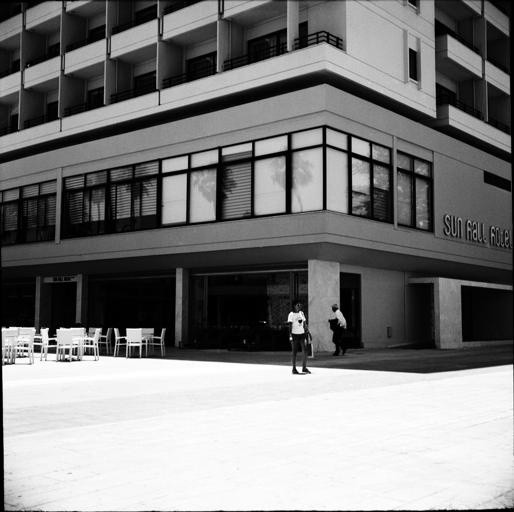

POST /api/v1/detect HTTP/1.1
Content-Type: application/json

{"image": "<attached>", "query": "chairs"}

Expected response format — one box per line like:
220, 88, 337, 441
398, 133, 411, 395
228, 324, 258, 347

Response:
2, 324, 167, 366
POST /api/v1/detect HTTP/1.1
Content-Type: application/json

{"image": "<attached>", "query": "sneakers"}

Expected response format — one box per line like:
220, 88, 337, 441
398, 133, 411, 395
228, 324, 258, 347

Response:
292, 368, 311, 374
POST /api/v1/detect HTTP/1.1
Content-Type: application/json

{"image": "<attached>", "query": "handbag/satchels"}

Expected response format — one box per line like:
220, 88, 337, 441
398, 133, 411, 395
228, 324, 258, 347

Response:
306, 332, 314, 358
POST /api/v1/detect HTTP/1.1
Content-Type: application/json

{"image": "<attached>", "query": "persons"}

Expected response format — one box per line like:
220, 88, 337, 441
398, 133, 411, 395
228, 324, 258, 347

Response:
328, 304, 347, 355
287, 300, 312, 374
